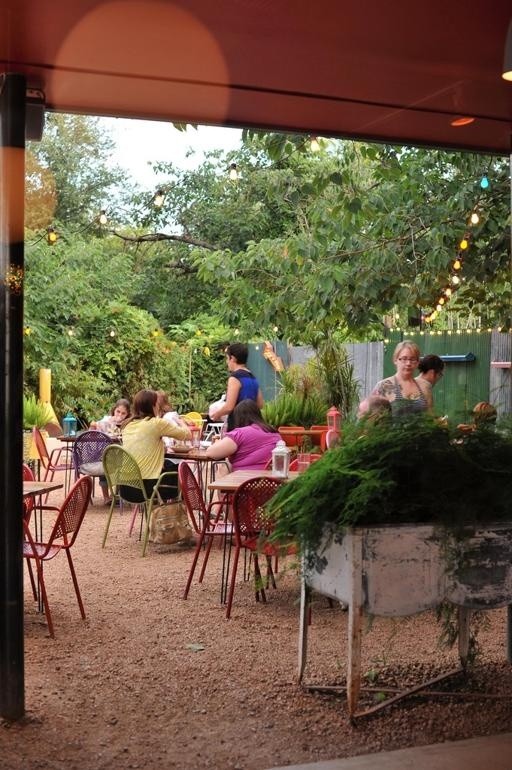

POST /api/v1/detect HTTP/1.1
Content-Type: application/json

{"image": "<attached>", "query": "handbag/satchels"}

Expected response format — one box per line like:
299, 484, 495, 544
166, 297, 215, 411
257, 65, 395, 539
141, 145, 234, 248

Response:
149, 502, 198, 544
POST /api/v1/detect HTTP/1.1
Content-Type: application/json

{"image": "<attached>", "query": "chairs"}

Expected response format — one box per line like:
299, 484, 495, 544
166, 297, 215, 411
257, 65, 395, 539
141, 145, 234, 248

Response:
178, 415, 194, 426
226, 478, 333, 624
178, 462, 261, 604
25, 463, 36, 524
205, 423, 223, 437
73, 430, 114, 506
312, 425, 334, 445
32, 426, 75, 504
185, 411, 202, 427
101, 445, 179, 557
24, 475, 93, 638
278, 427, 305, 447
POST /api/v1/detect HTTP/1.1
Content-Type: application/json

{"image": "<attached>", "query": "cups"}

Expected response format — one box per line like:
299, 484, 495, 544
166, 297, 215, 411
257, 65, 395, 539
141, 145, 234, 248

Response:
115, 417, 122, 438
297, 453, 310, 476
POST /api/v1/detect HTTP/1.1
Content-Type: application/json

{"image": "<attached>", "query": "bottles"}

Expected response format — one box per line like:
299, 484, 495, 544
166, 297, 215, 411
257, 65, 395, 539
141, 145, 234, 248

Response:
271, 440, 290, 479
327, 405, 341, 430
63, 412, 77, 439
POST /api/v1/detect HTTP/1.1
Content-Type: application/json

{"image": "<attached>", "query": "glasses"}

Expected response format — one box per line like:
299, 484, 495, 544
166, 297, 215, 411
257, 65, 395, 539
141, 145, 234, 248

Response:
398, 357, 415, 364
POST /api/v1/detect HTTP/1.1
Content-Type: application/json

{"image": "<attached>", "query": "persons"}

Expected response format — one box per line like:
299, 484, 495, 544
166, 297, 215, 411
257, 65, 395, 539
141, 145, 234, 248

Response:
364, 338, 448, 427
154, 390, 193, 468
120, 391, 211, 547
88, 399, 130, 507
211, 342, 263, 432
414, 354, 445, 389
206, 399, 282, 547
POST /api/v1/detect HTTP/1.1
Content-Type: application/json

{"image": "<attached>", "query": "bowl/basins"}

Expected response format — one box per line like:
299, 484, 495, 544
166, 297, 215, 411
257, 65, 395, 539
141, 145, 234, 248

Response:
201, 442, 212, 448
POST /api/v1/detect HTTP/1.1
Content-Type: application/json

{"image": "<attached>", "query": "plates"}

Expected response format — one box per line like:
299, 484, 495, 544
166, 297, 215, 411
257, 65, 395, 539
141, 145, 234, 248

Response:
170, 446, 194, 452
